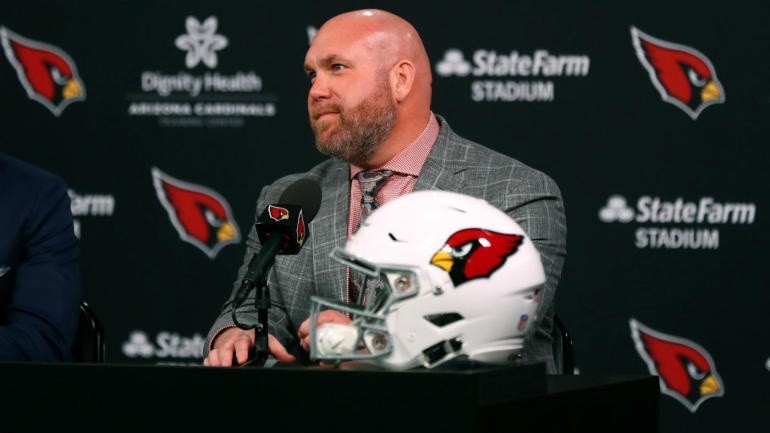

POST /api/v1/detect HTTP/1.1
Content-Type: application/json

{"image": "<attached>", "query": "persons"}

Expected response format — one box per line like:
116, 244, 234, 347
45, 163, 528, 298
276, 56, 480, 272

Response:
0, 151, 82, 361
202, 10, 568, 375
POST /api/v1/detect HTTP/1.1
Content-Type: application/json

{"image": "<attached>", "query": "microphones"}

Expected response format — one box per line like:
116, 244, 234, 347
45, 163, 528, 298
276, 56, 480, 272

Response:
232, 178, 324, 307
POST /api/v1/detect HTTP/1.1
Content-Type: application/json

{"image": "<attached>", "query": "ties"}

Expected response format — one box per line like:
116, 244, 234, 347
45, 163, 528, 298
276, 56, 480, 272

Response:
348, 170, 395, 317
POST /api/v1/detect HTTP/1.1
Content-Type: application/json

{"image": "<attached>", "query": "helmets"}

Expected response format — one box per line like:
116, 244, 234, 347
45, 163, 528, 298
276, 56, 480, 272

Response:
308, 190, 546, 375
